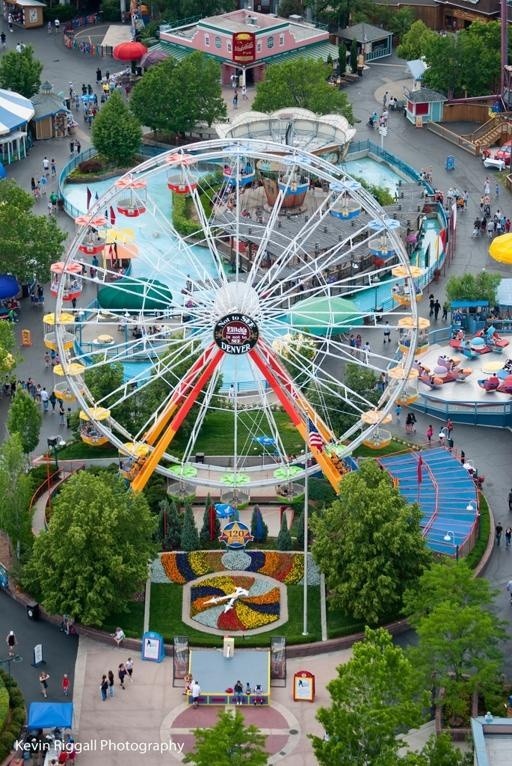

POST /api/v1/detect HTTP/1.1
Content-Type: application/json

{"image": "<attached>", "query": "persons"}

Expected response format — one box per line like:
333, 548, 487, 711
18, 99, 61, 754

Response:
233, 680, 244, 706
366, 90, 404, 133
0, 12, 60, 54
504, 527, 512, 548
349, 294, 512, 386
254, 685, 264, 705
124, 657, 136, 681
118, 663, 130, 690
100, 674, 109, 701
228, 71, 249, 108
505, 576, 512, 604
62, 65, 147, 137
508, 488, 512, 510
39, 671, 51, 701
60, 673, 71, 697
12, 726, 76, 765
392, 403, 483, 492
108, 671, 115, 697
246, 682, 251, 693
60, 614, 75, 634
113, 627, 125, 645
0, 139, 111, 425
416, 164, 512, 240
183, 673, 201, 709
495, 522, 503, 546
5, 630, 18, 658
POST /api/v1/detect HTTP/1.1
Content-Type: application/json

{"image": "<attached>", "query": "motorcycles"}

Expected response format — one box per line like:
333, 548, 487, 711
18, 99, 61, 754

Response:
367, 111, 377, 129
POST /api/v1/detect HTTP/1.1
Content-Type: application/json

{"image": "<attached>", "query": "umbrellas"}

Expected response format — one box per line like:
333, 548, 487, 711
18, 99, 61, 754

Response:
27, 700, 74, 730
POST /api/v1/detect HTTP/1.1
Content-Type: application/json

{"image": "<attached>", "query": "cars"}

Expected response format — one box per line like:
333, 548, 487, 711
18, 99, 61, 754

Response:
494, 140, 511, 165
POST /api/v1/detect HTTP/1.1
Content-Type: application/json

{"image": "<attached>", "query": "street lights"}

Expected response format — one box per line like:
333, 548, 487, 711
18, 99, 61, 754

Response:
54, 435, 66, 466
0, 654, 24, 695
439, 426, 453, 448
465, 500, 481, 540
444, 530, 459, 561
462, 460, 478, 476
